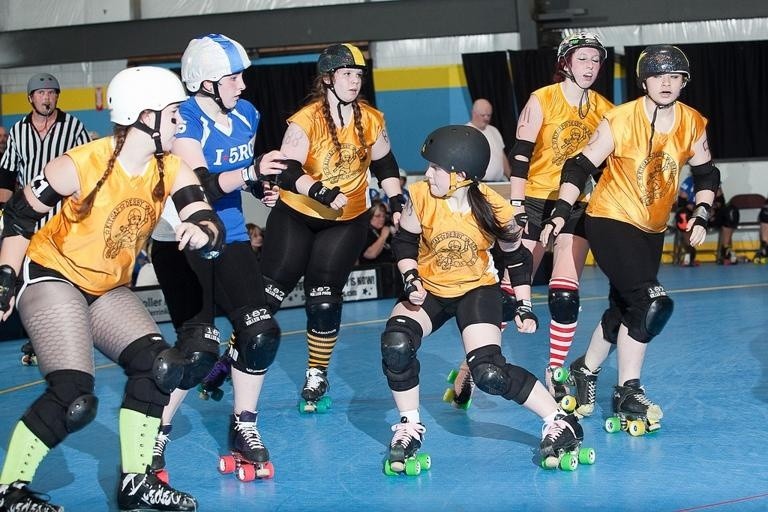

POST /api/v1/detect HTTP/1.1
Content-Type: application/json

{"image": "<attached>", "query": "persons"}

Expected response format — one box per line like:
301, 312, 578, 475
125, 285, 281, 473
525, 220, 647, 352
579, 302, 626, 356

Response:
151, 33, 280, 474
203, 43, 406, 402
245, 222, 265, 253
465, 97, 511, 183
356, 199, 394, 261
380, 122, 585, 459
454, 32, 616, 402
377, 165, 409, 214
539, 42, 720, 420
1, 72, 93, 366
0, 64, 227, 510
758, 198, 768, 255
677, 174, 740, 264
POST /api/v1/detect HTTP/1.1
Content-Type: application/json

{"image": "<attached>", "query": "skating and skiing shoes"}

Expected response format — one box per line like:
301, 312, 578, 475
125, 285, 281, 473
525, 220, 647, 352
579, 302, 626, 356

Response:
217, 409, 274, 482
442, 356, 483, 411
604, 378, 664, 438
20, 333, 58, 367
553, 353, 602, 418
145, 417, 173, 485
539, 411, 596, 471
679, 244, 700, 268
384, 415, 432, 477
754, 240, 768, 264
716, 243, 739, 266
299, 366, 334, 414
198, 346, 254, 402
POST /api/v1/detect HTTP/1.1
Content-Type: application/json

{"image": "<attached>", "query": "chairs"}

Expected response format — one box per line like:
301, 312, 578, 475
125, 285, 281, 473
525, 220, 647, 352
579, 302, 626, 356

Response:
665, 200, 722, 265
729, 193, 765, 253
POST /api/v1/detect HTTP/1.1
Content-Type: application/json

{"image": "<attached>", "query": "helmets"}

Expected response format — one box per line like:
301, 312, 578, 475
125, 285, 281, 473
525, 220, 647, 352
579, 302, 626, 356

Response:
557, 32, 608, 64
26, 71, 62, 96
180, 33, 253, 93
106, 65, 188, 127
421, 124, 492, 182
318, 42, 366, 75
636, 43, 691, 81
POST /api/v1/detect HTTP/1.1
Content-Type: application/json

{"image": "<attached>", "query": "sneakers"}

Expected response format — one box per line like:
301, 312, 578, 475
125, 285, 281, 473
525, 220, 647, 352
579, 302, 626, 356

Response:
0, 479, 66, 511
117, 464, 200, 511
